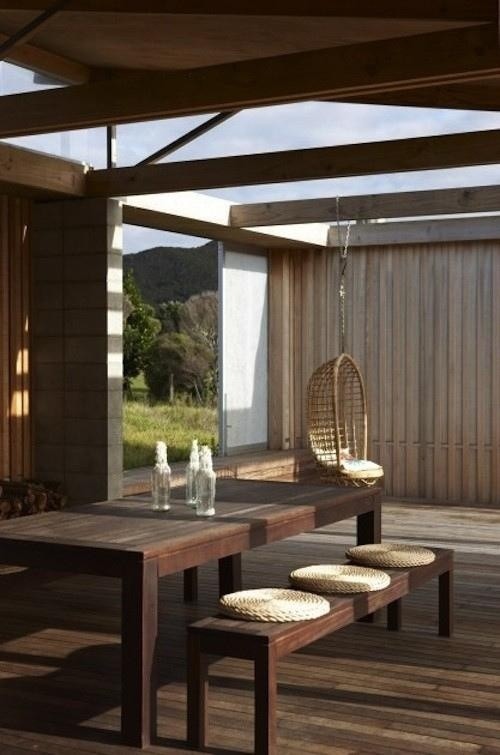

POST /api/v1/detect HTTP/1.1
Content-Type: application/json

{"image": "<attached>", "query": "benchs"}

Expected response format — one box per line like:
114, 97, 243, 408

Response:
182, 543, 455, 755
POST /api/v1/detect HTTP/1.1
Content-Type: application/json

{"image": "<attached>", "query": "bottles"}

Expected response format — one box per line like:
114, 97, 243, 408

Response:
194, 444, 217, 517
152, 441, 172, 511
185, 439, 200, 506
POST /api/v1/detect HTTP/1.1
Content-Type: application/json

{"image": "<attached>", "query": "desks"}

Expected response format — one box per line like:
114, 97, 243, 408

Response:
0, 474, 383, 750
